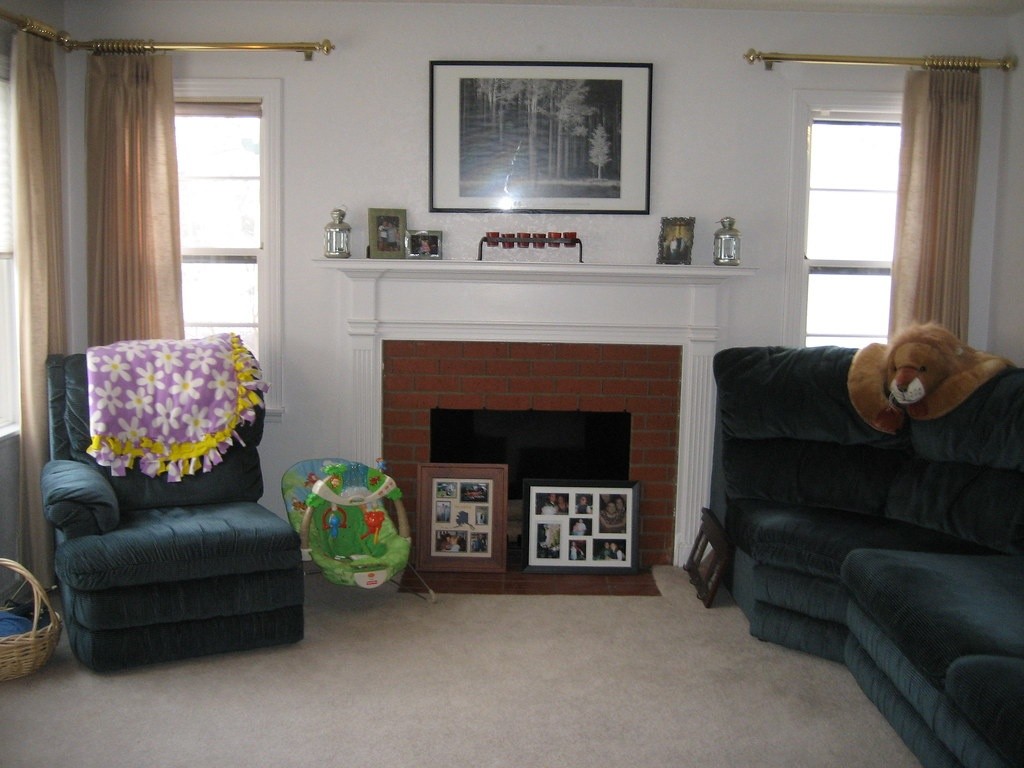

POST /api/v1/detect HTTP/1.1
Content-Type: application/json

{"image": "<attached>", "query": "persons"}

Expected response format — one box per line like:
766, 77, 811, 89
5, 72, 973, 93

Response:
669, 231, 685, 260
377, 221, 398, 250
434, 482, 629, 563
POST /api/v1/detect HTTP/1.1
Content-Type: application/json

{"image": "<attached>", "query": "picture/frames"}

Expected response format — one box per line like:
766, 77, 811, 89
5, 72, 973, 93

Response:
522, 479, 639, 576
366, 208, 443, 260
416, 463, 507, 573
657, 215, 695, 266
429, 60, 652, 215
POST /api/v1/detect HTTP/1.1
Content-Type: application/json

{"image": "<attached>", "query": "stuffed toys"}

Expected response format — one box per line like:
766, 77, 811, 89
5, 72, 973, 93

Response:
847, 323, 1017, 434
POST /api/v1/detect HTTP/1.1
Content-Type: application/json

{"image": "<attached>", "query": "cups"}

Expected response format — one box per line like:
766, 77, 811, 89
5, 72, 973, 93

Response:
517, 233, 531, 248
501, 234, 515, 248
548, 232, 561, 248
533, 234, 546, 248
564, 232, 576, 247
486, 232, 499, 247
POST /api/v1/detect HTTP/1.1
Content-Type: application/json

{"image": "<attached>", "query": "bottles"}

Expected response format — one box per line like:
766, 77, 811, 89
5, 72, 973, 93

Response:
713, 217, 740, 265
323, 209, 351, 258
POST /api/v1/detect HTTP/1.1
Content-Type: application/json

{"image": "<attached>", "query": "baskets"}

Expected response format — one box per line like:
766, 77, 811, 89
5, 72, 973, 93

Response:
0, 558, 63, 683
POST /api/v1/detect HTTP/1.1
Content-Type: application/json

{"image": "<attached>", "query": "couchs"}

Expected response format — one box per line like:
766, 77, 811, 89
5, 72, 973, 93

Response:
712, 346, 1024, 768
42, 333, 304, 673
